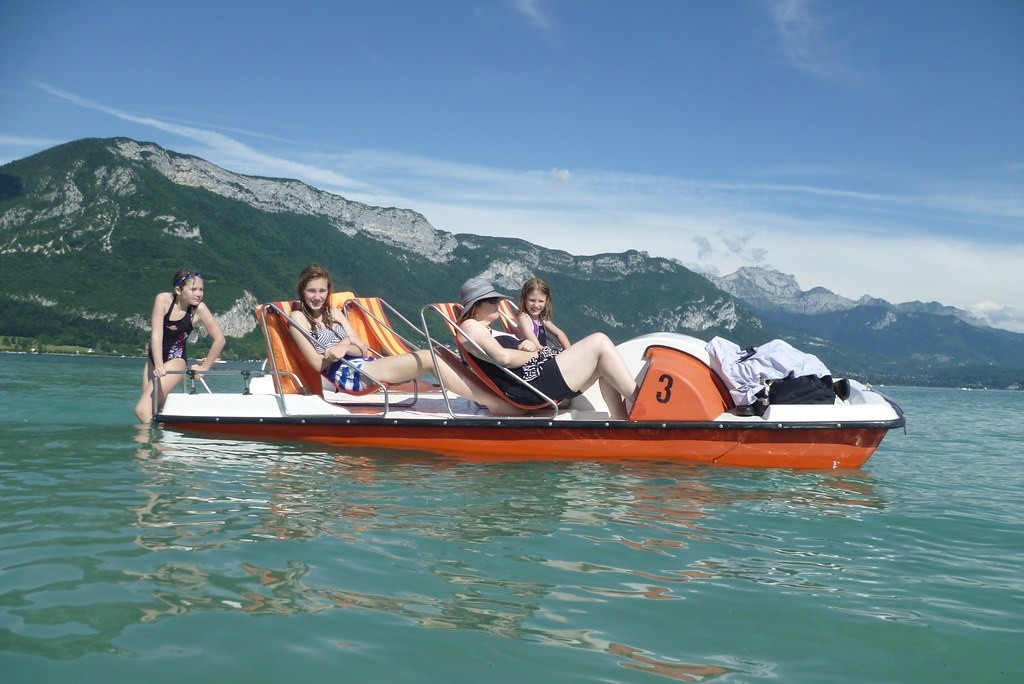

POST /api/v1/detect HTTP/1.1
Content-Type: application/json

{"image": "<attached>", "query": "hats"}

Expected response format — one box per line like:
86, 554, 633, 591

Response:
458, 278, 514, 321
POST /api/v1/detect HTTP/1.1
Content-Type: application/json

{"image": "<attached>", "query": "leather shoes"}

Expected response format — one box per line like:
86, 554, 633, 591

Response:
737, 404, 754, 415
833, 377, 851, 401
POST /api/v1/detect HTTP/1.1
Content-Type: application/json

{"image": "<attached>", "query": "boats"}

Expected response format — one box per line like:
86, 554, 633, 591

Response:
152, 291, 908, 470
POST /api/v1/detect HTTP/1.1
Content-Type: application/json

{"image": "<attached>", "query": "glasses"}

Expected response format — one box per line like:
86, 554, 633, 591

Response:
477, 298, 499, 304
176, 273, 206, 285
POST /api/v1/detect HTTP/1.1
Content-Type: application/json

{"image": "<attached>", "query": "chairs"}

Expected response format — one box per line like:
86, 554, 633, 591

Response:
342, 297, 462, 392
494, 298, 564, 350
260, 301, 419, 419
421, 298, 572, 420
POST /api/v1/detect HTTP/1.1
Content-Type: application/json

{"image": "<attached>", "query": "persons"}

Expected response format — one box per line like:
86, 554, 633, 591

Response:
457, 278, 640, 421
135, 268, 225, 424
517, 278, 571, 351
289, 265, 530, 415
